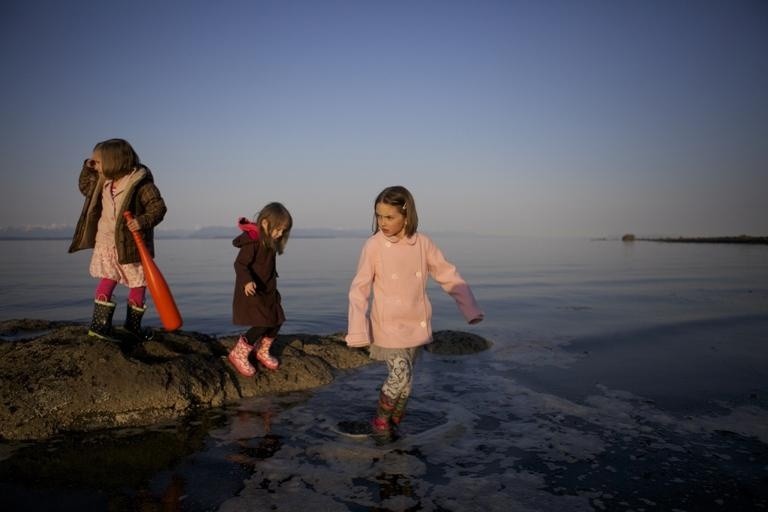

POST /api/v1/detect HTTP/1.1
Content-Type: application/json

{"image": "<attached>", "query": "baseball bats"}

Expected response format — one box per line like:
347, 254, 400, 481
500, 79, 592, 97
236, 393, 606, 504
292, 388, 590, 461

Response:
124, 211, 182, 332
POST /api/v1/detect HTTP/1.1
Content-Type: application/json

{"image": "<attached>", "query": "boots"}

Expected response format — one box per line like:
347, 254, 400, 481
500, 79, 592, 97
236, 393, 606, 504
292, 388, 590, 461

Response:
88, 299, 148, 341
368, 391, 409, 434
228, 335, 279, 377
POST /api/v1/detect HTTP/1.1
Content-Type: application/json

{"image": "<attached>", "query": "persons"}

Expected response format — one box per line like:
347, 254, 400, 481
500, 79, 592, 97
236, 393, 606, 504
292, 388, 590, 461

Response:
67, 139, 167, 344
227, 201, 293, 377
344, 185, 483, 446
